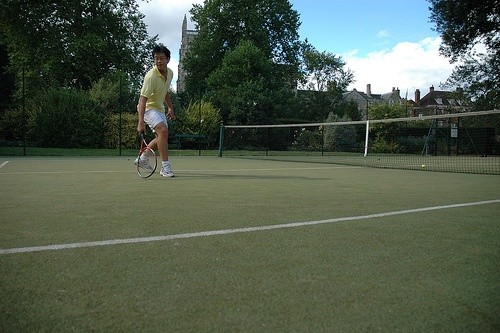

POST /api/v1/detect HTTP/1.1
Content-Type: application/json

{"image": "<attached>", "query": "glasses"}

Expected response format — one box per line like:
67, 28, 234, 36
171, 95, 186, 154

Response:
153, 57, 167, 61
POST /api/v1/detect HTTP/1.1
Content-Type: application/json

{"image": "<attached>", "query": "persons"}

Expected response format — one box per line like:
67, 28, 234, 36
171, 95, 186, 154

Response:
132, 45, 180, 178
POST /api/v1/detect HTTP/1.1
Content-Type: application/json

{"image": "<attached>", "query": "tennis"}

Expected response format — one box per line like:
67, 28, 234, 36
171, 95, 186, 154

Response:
421, 164, 425, 168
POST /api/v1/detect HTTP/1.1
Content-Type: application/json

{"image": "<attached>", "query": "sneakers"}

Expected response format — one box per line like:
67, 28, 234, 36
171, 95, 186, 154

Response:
134, 156, 154, 174
160, 163, 175, 177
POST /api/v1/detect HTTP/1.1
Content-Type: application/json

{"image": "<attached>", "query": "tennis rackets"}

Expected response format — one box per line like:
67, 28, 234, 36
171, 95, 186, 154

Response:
136, 131, 157, 178
